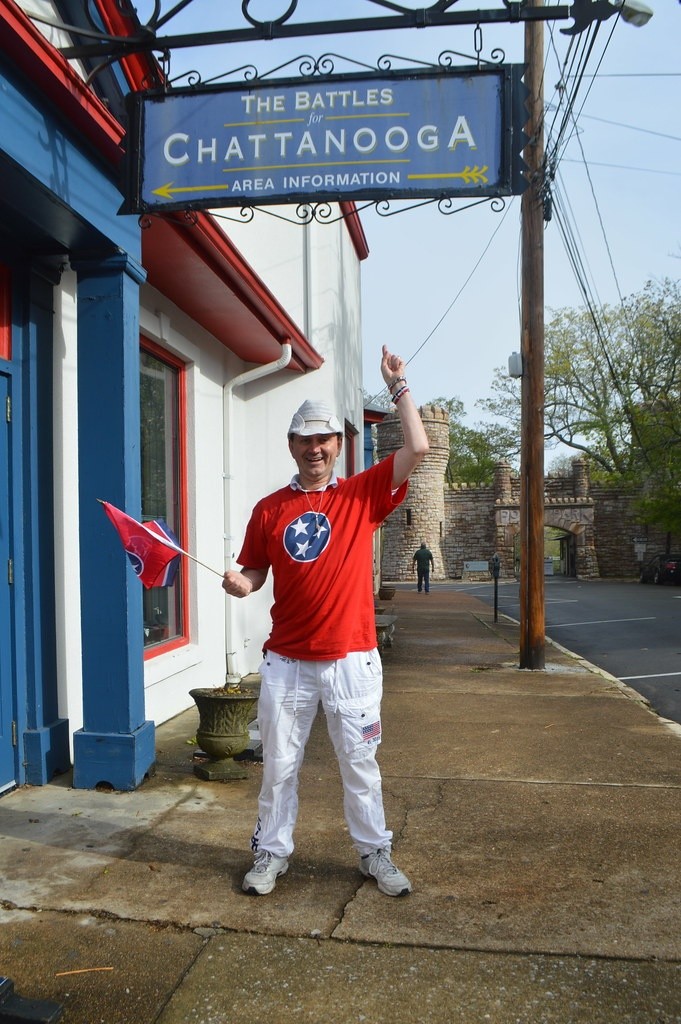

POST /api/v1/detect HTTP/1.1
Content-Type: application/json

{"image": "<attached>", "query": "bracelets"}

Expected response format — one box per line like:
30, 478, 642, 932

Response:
388, 375, 408, 391
390, 385, 411, 404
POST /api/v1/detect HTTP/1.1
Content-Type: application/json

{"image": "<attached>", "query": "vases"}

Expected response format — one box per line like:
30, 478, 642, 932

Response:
379, 587, 396, 600
383, 586, 396, 597
189, 688, 260, 780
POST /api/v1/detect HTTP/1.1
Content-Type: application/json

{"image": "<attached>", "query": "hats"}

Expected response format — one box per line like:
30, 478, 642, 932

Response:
288, 400, 342, 436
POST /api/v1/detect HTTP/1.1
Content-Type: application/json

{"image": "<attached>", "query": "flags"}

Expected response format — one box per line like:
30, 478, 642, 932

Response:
100, 499, 183, 592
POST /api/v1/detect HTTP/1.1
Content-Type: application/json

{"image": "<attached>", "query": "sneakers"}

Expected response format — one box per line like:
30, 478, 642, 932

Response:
242, 850, 289, 895
358, 850, 411, 897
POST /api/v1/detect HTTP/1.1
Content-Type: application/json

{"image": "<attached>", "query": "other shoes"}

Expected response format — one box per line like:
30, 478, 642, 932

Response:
417, 591, 422, 594
425, 591, 429, 595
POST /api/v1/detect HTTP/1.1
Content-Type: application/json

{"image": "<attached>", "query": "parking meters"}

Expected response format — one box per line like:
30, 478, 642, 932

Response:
488, 553, 501, 623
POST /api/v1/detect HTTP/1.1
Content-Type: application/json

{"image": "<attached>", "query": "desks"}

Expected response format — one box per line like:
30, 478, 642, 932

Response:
375, 614, 398, 658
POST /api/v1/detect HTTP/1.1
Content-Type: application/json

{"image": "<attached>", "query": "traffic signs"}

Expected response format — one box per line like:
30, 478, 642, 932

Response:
631, 536, 648, 542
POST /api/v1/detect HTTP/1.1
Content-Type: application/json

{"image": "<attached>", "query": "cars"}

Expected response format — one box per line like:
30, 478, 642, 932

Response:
639, 553, 681, 584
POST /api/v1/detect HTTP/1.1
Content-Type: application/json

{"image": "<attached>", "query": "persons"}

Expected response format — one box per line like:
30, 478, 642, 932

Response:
411, 541, 436, 595
219, 345, 428, 898
